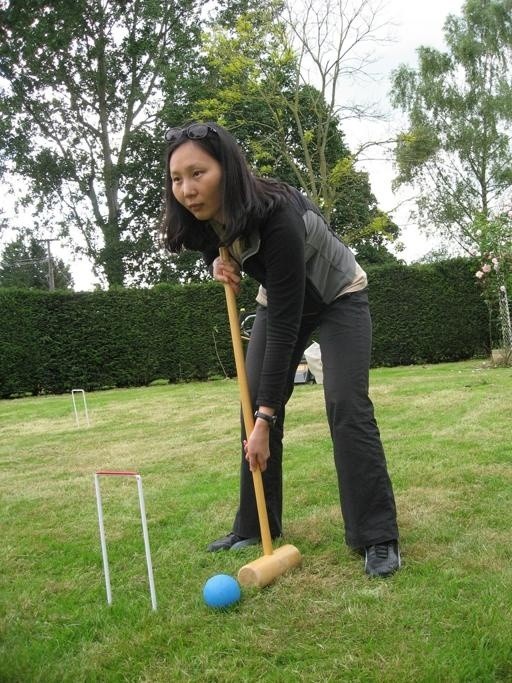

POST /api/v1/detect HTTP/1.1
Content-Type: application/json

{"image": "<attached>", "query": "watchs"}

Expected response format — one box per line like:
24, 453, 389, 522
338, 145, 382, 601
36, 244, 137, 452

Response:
254, 411, 277, 430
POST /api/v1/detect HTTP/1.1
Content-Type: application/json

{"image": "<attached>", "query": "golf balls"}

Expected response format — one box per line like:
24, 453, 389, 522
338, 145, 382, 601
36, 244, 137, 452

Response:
203, 574, 240, 607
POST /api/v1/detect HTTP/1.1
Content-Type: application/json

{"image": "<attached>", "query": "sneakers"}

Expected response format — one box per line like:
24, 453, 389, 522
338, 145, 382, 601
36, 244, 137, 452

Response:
207, 532, 261, 552
365, 540, 400, 578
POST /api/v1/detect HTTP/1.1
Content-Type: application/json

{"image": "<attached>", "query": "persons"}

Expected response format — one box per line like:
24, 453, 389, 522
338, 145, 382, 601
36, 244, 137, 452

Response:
161, 122, 401, 581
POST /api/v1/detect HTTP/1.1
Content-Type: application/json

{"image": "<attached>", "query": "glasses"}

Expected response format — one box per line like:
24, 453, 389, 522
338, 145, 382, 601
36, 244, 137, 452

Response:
164, 124, 216, 145
